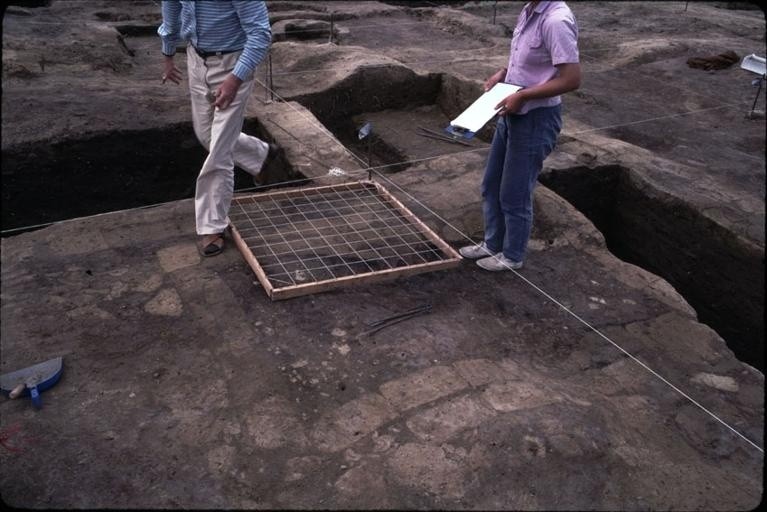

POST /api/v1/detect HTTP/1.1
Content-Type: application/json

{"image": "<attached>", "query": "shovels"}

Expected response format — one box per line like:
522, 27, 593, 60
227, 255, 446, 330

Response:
8, 375, 41, 399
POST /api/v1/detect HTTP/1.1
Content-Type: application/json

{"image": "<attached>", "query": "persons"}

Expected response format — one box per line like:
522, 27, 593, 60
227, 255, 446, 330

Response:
157, 0, 280, 256
458, 0, 580, 272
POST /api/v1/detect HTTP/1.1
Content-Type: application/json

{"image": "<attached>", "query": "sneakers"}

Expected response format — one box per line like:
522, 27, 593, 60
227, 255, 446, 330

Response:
475, 251, 523, 272
459, 242, 494, 259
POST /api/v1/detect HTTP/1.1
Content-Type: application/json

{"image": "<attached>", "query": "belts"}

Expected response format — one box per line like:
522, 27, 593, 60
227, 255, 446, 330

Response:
191, 47, 242, 58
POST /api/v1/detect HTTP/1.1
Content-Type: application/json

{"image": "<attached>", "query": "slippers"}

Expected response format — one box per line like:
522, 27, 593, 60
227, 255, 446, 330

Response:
250, 142, 282, 187
200, 233, 227, 257
